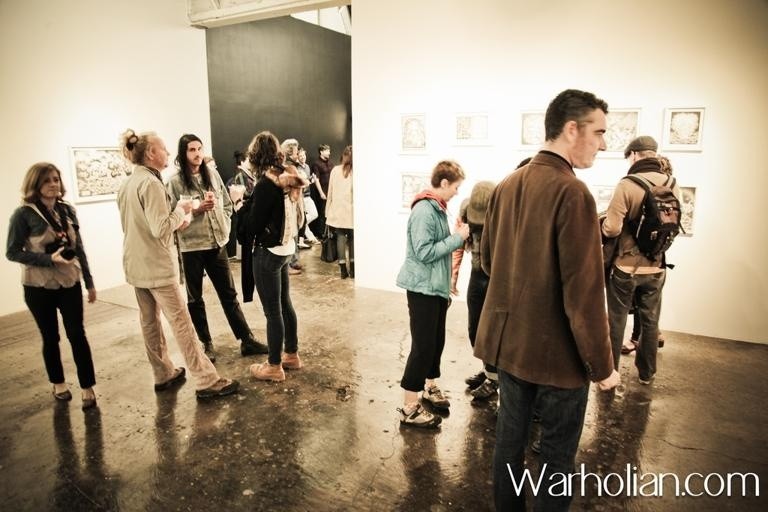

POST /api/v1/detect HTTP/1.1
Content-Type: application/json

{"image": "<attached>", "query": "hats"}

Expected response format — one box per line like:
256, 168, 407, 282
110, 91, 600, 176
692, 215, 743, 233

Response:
623, 135, 657, 155
466, 180, 495, 226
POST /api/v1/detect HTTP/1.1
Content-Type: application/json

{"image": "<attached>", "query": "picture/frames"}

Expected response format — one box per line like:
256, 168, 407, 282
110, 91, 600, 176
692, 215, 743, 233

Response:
399, 111, 429, 155
68, 143, 134, 204
519, 109, 548, 150
397, 169, 433, 214
594, 106, 642, 159
450, 111, 496, 147
591, 184, 616, 215
661, 105, 706, 152
673, 182, 698, 236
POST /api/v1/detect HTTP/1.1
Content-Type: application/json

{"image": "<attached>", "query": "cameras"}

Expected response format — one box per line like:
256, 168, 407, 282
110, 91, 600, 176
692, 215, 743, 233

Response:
44, 237, 75, 261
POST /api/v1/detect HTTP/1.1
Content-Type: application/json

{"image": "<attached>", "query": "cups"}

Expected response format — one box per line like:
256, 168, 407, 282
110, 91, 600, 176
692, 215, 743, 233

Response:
205, 191, 214, 211
180, 194, 192, 201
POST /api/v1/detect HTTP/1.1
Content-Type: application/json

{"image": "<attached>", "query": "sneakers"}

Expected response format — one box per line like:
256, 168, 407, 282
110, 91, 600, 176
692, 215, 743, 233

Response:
287, 239, 313, 274
421, 386, 451, 409
638, 373, 655, 385
195, 378, 239, 398
396, 401, 442, 430
154, 367, 185, 393
204, 342, 216, 363
240, 340, 301, 382
620, 340, 639, 354
465, 369, 499, 399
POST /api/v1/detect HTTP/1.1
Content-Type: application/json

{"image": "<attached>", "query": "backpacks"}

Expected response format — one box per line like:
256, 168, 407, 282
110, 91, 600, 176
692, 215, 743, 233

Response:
620, 173, 681, 258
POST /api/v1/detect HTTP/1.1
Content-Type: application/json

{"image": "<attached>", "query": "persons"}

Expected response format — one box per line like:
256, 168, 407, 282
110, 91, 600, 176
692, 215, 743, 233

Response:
5, 162, 97, 411
396, 90, 683, 512
115, 129, 354, 401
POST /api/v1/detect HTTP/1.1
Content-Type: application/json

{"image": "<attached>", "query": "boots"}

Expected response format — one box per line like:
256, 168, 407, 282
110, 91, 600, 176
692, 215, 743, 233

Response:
338, 262, 354, 278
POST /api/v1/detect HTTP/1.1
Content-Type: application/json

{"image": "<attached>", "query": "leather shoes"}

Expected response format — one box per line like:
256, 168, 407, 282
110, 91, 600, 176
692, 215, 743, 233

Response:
81, 391, 97, 409
52, 384, 72, 401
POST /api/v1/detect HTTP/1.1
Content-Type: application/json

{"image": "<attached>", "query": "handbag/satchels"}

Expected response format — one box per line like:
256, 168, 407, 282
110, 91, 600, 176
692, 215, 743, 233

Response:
320, 233, 338, 263
